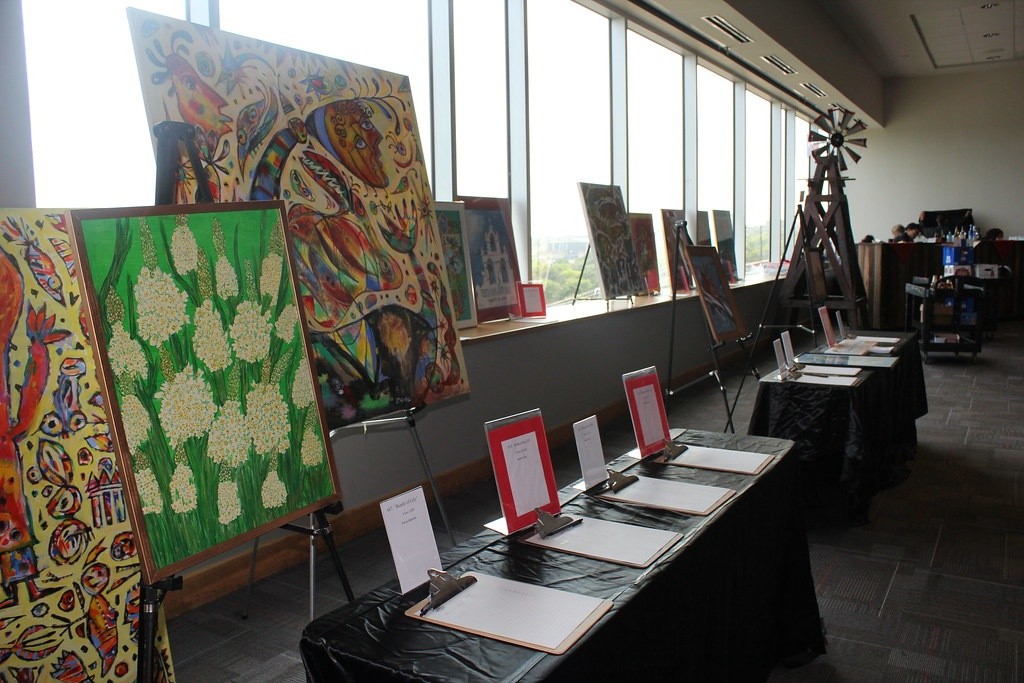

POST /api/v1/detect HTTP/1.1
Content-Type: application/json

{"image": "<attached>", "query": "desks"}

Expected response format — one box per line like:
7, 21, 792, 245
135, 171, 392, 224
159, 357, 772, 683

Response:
747, 331, 929, 539
298, 427, 828, 683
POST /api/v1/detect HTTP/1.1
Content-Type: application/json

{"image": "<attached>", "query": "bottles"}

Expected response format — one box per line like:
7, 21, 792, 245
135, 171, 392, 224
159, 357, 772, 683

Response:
946, 224, 979, 243
929, 274, 954, 289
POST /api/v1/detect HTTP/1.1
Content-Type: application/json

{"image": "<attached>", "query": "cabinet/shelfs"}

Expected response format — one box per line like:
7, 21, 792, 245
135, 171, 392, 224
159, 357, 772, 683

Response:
905, 275, 991, 364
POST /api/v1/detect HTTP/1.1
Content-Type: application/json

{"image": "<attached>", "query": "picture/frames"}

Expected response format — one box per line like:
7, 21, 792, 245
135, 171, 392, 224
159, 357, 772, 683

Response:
433, 201, 478, 329
63, 201, 343, 588
683, 244, 748, 345
457, 195, 523, 323
629, 213, 660, 294
